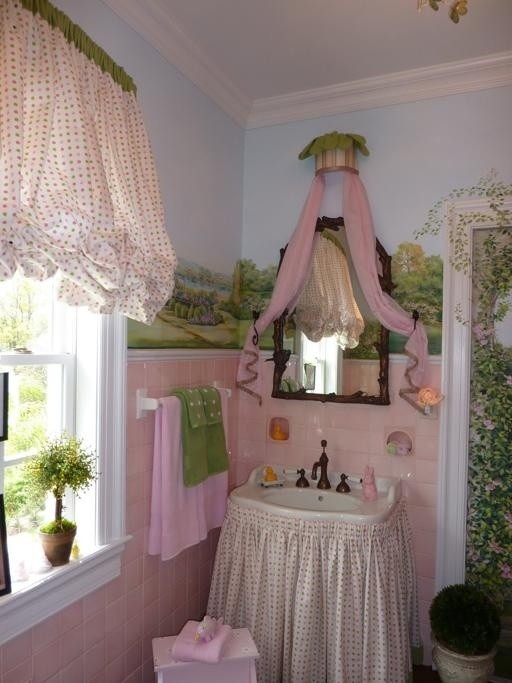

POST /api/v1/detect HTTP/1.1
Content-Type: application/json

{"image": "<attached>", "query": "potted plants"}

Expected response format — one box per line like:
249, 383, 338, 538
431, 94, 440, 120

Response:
23, 428, 102, 567
428, 584, 501, 683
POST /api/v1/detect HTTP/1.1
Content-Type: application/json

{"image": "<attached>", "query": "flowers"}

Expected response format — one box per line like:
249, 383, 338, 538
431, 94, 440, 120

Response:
412, 165, 512, 606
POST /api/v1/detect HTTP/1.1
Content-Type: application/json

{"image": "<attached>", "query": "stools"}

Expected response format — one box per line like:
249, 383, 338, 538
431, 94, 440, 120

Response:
151, 628, 260, 683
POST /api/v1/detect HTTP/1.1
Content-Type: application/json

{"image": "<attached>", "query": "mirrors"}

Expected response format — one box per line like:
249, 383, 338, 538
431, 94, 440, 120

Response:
271, 216, 391, 405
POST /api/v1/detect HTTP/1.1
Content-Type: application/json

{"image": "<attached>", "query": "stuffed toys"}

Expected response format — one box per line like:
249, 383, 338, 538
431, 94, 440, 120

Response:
192, 615, 225, 641
359, 464, 379, 501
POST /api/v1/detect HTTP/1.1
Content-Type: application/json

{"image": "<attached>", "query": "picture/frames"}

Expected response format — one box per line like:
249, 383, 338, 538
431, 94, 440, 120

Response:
0, 494, 11, 597
0, 373, 8, 441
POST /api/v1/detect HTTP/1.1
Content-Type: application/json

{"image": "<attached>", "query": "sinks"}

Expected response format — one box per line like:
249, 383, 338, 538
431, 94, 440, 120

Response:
230, 463, 401, 524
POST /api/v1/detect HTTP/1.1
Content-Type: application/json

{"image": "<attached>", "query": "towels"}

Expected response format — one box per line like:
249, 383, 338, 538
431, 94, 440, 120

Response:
172, 620, 233, 663
148, 390, 229, 562
172, 387, 229, 487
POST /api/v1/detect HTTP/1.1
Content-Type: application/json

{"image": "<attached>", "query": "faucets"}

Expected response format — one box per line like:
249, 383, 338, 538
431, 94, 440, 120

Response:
311, 439, 331, 489
